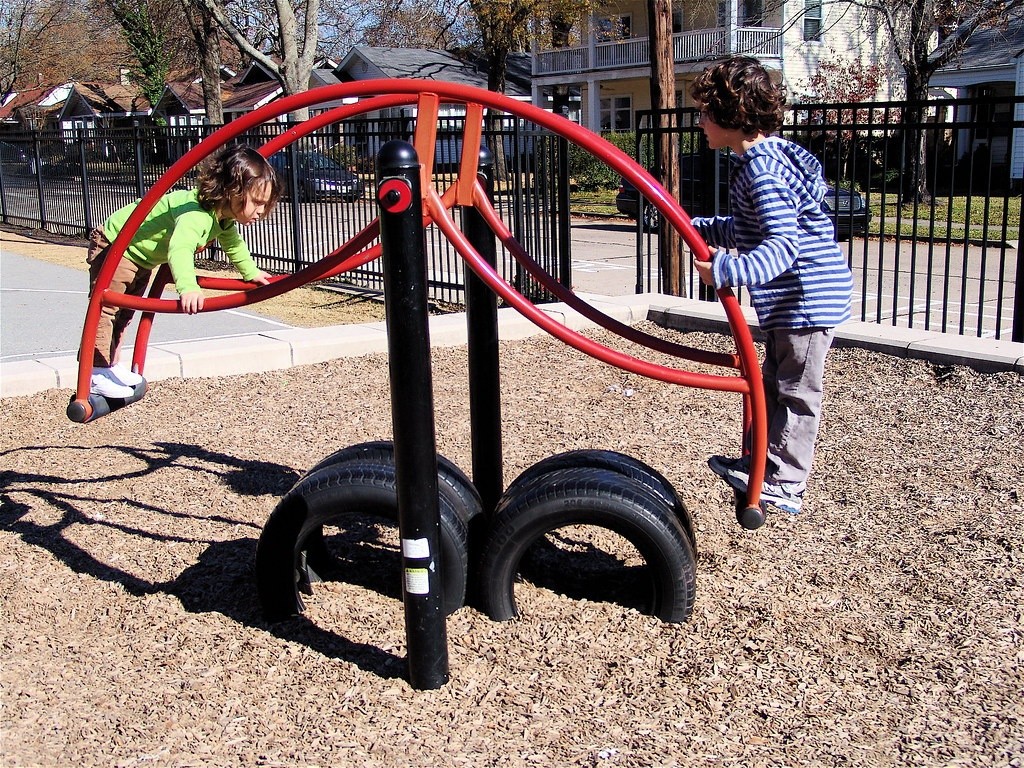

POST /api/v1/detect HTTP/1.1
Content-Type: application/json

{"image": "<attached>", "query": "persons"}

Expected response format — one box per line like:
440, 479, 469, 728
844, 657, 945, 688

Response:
77, 144, 285, 398
676, 57, 850, 511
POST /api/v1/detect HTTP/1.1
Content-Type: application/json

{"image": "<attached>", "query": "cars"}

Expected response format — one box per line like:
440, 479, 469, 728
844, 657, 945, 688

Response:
614, 150, 876, 243
266, 150, 365, 204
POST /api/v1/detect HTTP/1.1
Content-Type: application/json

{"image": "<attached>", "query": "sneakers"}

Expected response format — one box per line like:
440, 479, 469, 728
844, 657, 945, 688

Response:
707, 455, 802, 515
88, 364, 142, 398
